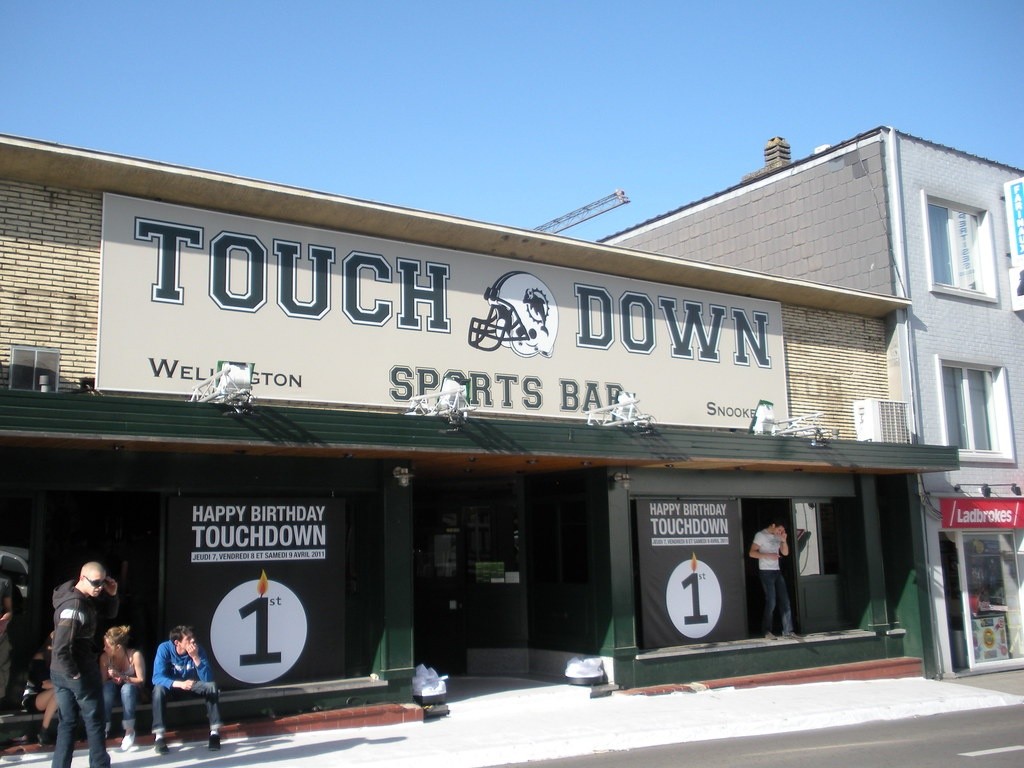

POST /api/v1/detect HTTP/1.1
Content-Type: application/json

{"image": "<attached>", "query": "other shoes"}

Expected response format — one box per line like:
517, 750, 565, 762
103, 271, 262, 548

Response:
783, 631, 805, 641
153, 739, 171, 755
764, 631, 778, 640
209, 733, 221, 751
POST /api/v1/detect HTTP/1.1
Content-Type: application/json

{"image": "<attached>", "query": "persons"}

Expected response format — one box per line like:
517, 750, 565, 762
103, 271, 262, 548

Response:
50, 560, 120, 768
100, 625, 151, 749
151, 624, 222, 755
0, 575, 12, 698
749, 519, 800, 640
22, 630, 58, 745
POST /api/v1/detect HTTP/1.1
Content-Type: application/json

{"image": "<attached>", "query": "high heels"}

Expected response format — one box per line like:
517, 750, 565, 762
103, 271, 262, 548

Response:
36, 731, 52, 747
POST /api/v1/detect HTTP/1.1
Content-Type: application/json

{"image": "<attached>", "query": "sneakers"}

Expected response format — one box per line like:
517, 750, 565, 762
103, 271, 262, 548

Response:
121, 731, 135, 751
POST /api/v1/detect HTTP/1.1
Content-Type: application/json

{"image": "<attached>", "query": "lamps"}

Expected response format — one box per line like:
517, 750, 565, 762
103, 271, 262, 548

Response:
186, 360, 255, 417
393, 466, 414, 486
988, 483, 1021, 495
585, 392, 656, 436
774, 412, 840, 446
749, 399, 775, 435
437, 377, 472, 402
406, 379, 475, 430
955, 483, 991, 498
609, 472, 631, 490
69, 379, 94, 395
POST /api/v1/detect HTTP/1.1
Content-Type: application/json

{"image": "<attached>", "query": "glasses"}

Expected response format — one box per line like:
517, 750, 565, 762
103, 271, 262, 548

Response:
83, 575, 108, 589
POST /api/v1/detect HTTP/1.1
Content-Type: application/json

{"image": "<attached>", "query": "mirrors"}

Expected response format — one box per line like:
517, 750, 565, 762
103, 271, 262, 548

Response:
795, 502, 820, 576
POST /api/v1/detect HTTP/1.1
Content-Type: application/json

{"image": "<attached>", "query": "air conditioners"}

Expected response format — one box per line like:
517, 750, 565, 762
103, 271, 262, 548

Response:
854, 399, 910, 443
